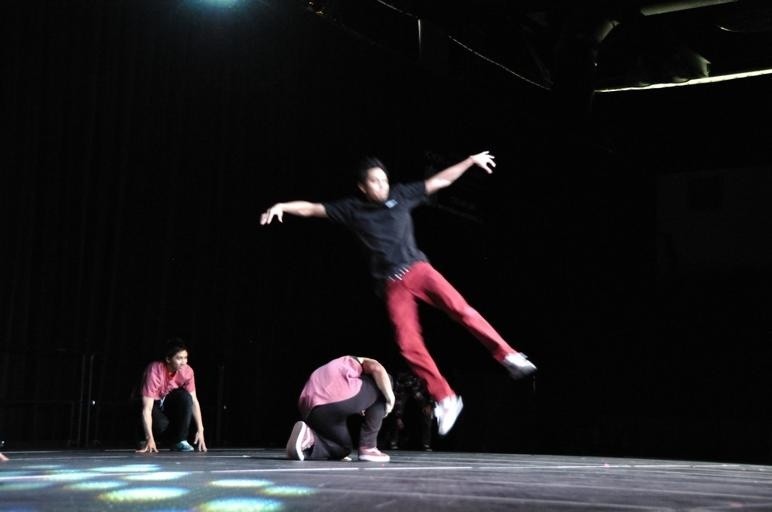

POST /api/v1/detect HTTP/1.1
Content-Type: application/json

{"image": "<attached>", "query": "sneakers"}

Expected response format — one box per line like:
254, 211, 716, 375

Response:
502, 352, 537, 378
173, 440, 194, 452
434, 395, 463, 435
358, 447, 390, 462
391, 442, 399, 449
287, 421, 314, 461
424, 444, 433, 451
137, 441, 146, 448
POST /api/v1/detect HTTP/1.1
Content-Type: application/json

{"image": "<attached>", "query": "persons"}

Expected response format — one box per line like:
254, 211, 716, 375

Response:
387, 357, 437, 453
131, 342, 209, 453
286, 354, 396, 463
260, 147, 538, 434
